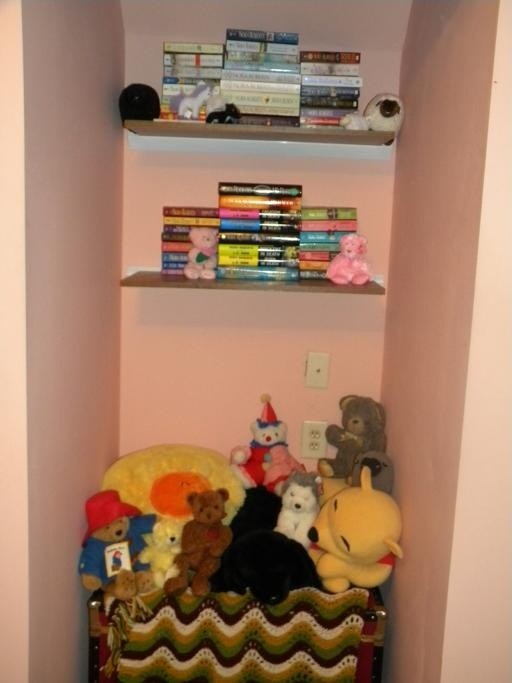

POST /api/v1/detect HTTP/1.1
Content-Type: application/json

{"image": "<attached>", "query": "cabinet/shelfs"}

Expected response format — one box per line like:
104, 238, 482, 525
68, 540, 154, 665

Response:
121, 119, 396, 296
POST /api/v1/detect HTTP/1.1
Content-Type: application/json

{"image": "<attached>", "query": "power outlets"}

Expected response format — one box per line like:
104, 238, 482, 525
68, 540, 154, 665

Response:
302, 421, 327, 460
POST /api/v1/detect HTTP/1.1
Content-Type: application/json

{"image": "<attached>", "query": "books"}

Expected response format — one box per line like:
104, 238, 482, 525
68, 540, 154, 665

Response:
153, 27, 362, 130
158, 181, 359, 282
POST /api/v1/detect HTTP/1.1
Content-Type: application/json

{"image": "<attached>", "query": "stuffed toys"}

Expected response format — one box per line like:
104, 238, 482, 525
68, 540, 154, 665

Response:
77, 392, 403, 606
230, 394, 287, 489
317, 395, 387, 486
338, 92, 405, 146
326, 234, 371, 287
77, 444, 246, 601
209, 470, 403, 604
262, 445, 307, 497
